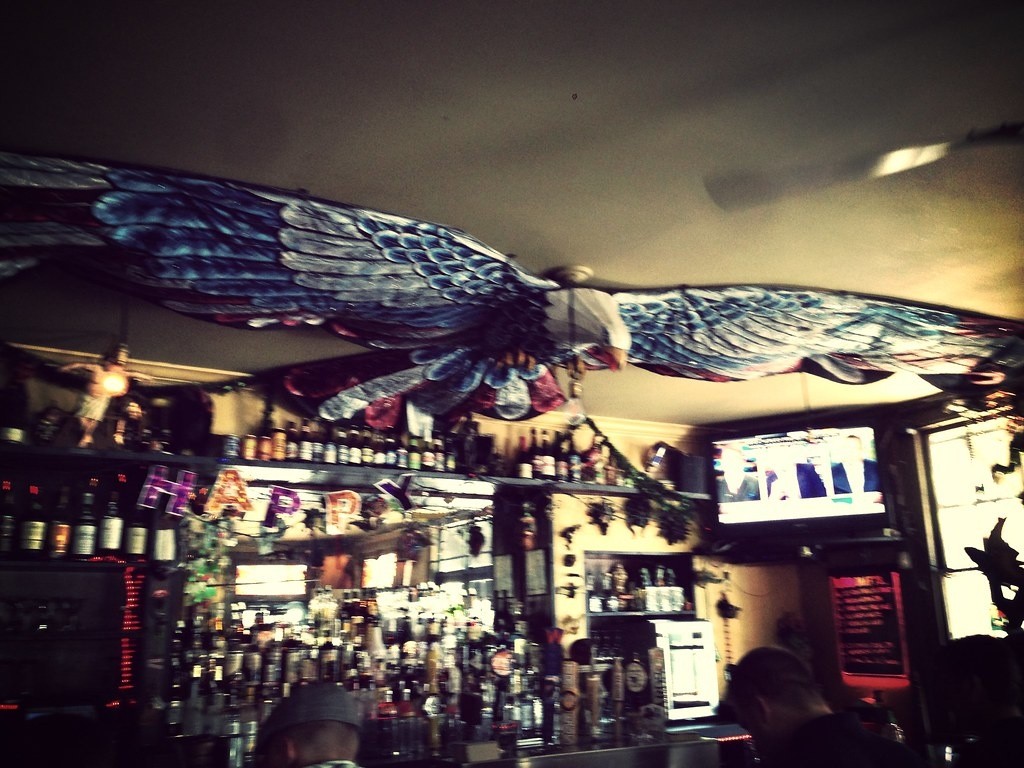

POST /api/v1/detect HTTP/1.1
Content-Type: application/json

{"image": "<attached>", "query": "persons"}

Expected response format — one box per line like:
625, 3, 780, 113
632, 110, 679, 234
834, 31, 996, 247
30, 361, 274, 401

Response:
708, 610, 1024, 768
252, 681, 361, 768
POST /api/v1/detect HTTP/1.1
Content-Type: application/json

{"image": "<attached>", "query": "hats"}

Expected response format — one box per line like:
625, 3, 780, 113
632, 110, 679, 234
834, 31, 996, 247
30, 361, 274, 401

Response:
256, 684, 363, 742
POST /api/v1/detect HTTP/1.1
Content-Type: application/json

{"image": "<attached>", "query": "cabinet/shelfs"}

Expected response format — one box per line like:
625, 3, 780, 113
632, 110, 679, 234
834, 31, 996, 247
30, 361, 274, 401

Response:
0, 436, 755, 768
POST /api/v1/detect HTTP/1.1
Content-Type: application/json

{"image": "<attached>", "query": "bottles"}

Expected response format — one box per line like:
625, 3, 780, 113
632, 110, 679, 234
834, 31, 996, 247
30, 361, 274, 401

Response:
1, 382, 671, 768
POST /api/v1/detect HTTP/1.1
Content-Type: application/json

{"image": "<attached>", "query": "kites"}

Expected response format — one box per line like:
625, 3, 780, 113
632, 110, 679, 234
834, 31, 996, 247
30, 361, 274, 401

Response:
0, 154, 1024, 447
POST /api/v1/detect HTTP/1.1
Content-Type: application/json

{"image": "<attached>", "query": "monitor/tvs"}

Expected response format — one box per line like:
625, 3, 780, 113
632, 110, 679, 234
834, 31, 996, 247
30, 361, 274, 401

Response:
706, 422, 890, 544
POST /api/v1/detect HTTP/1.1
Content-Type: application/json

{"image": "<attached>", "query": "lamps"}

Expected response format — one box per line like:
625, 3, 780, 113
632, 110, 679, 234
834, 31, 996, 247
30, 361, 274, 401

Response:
553, 264, 594, 424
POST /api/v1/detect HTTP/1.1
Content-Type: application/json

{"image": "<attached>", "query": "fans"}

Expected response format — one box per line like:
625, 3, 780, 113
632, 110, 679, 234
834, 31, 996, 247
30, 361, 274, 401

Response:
701, 12, 1024, 233
